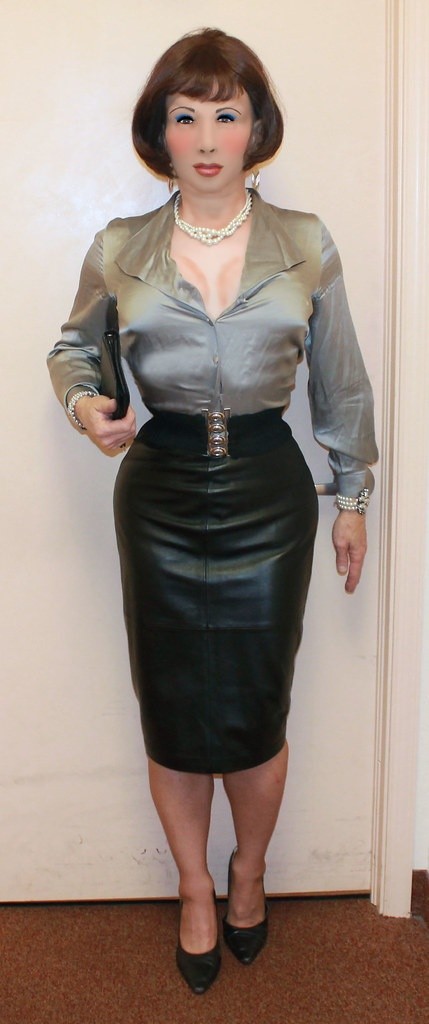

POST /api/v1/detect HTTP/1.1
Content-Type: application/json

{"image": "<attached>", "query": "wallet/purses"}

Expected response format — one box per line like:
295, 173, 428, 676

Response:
98, 331, 130, 419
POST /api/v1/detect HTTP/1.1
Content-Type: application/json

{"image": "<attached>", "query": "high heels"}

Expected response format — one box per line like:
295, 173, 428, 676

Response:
223, 845, 269, 964
175, 877, 221, 994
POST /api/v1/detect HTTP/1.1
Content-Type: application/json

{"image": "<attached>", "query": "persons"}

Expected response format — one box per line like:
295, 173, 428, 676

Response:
46, 28, 379, 994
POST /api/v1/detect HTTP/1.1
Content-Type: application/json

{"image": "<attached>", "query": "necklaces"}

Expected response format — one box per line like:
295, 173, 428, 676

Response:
173, 189, 252, 246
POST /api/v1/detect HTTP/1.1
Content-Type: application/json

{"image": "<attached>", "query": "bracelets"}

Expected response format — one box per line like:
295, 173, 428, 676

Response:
68, 391, 100, 430
335, 488, 371, 515
